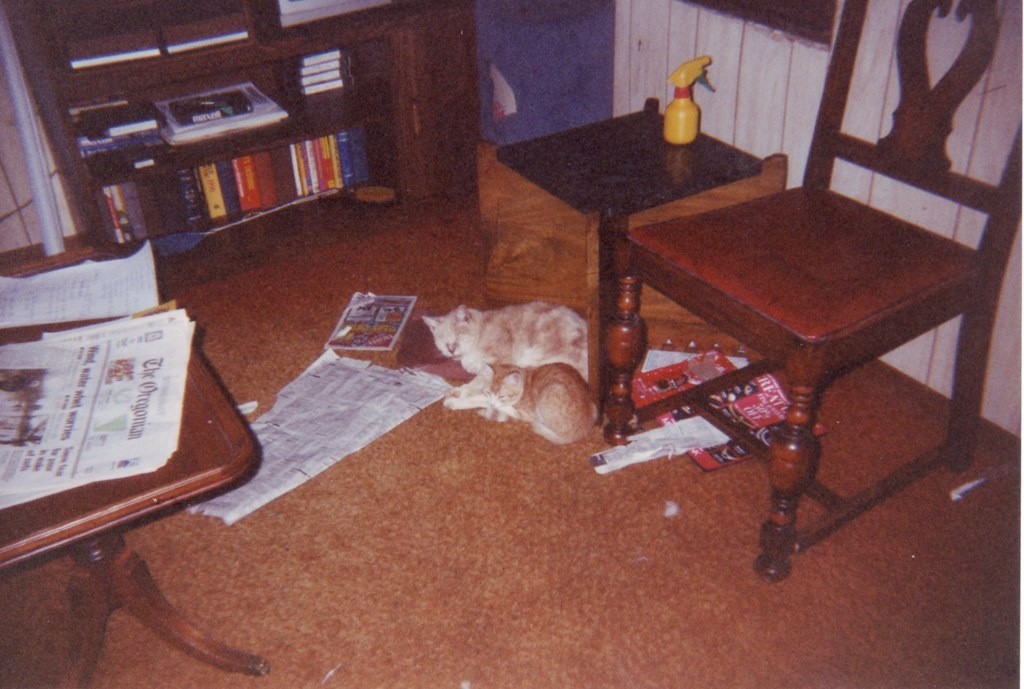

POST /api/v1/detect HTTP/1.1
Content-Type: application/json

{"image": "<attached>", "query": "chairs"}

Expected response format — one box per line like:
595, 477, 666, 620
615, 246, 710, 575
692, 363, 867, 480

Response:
603, 0, 1022, 585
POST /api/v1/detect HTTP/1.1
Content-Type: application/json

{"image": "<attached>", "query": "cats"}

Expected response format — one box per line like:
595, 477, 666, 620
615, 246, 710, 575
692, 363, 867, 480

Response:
478, 362, 594, 445
422, 301, 587, 406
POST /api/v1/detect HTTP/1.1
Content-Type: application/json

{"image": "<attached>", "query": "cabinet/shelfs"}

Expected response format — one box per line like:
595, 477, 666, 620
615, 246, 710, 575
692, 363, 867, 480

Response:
0, 0, 479, 299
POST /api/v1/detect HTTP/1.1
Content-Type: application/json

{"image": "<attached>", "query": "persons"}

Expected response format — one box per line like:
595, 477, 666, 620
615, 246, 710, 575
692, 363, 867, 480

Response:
0, 369, 48, 445
112, 359, 134, 378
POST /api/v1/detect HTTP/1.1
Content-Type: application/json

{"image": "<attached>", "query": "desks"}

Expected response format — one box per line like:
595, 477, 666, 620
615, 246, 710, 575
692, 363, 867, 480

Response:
0, 248, 269, 689
476, 97, 788, 424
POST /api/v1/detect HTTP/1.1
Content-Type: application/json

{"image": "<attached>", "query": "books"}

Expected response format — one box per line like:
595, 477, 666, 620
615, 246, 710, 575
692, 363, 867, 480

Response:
66, 12, 370, 244
